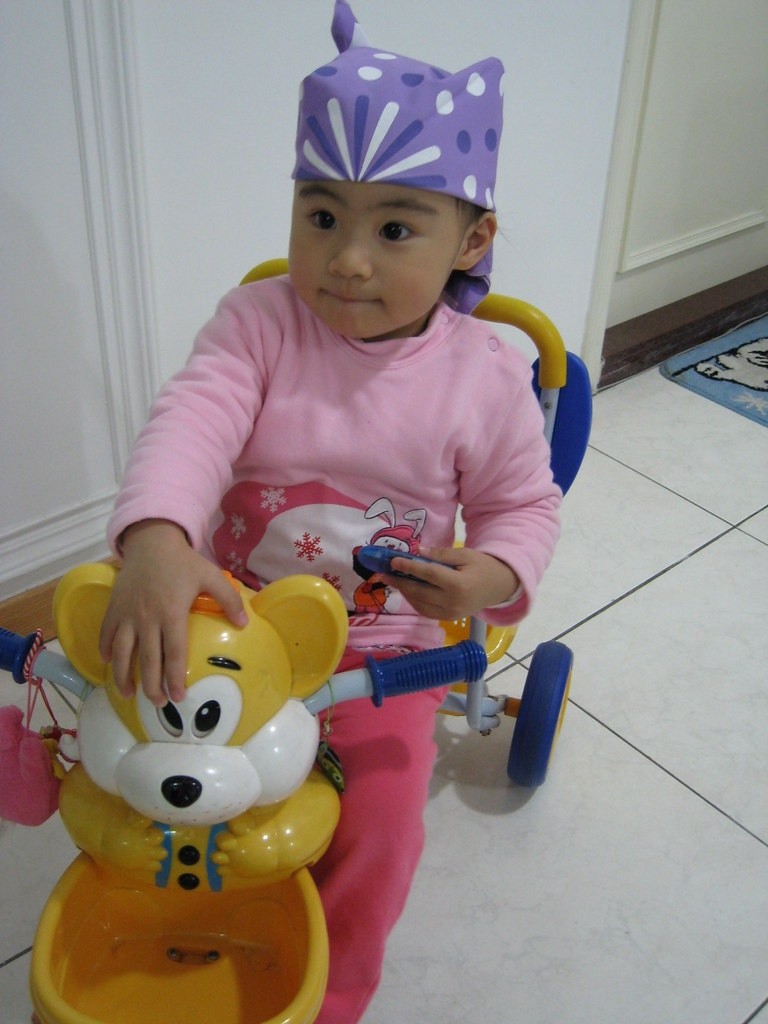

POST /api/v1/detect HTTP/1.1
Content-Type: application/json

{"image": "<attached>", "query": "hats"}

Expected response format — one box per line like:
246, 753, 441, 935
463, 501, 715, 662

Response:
292, 0, 504, 313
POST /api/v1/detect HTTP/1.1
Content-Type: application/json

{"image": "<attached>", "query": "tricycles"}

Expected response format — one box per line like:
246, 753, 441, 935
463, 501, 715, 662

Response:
0, 259, 595, 1022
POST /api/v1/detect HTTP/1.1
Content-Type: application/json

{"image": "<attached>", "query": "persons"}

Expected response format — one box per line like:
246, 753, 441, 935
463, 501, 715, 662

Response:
106, 48, 563, 1024
